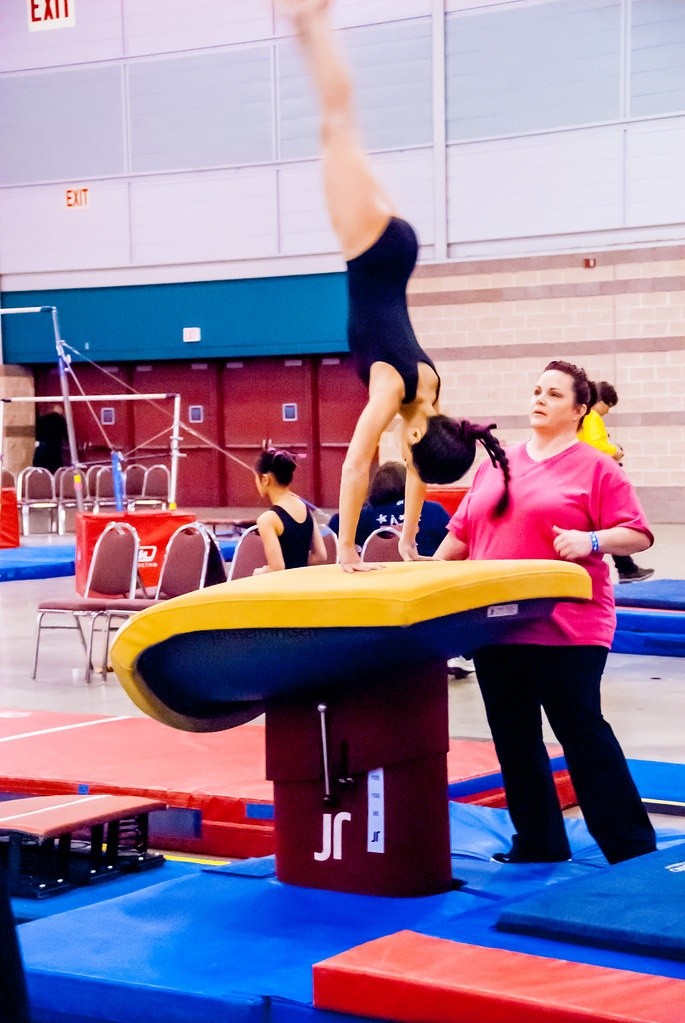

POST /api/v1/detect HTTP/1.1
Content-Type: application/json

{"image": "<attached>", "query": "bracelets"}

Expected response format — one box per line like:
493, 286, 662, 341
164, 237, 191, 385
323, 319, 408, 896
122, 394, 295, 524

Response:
589, 530, 598, 554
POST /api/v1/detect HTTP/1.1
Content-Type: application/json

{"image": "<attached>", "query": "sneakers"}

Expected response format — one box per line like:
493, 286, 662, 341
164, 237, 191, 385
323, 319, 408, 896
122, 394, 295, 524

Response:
619, 567, 654, 583
446, 653, 476, 672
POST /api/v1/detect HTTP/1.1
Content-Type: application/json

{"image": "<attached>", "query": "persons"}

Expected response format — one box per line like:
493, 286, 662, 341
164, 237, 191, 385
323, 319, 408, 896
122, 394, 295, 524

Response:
576, 381, 654, 585
330, 461, 451, 561
33, 404, 70, 470
433, 358, 657, 866
291, 1, 512, 576
251, 446, 327, 574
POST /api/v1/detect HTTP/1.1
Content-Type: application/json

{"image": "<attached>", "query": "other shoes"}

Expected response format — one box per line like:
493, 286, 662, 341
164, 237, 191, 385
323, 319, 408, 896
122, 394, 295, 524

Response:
491, 848, 574, 865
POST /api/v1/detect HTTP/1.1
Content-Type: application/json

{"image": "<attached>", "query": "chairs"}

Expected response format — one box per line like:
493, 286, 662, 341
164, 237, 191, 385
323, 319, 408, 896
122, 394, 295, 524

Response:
0, 463, 412, 684
